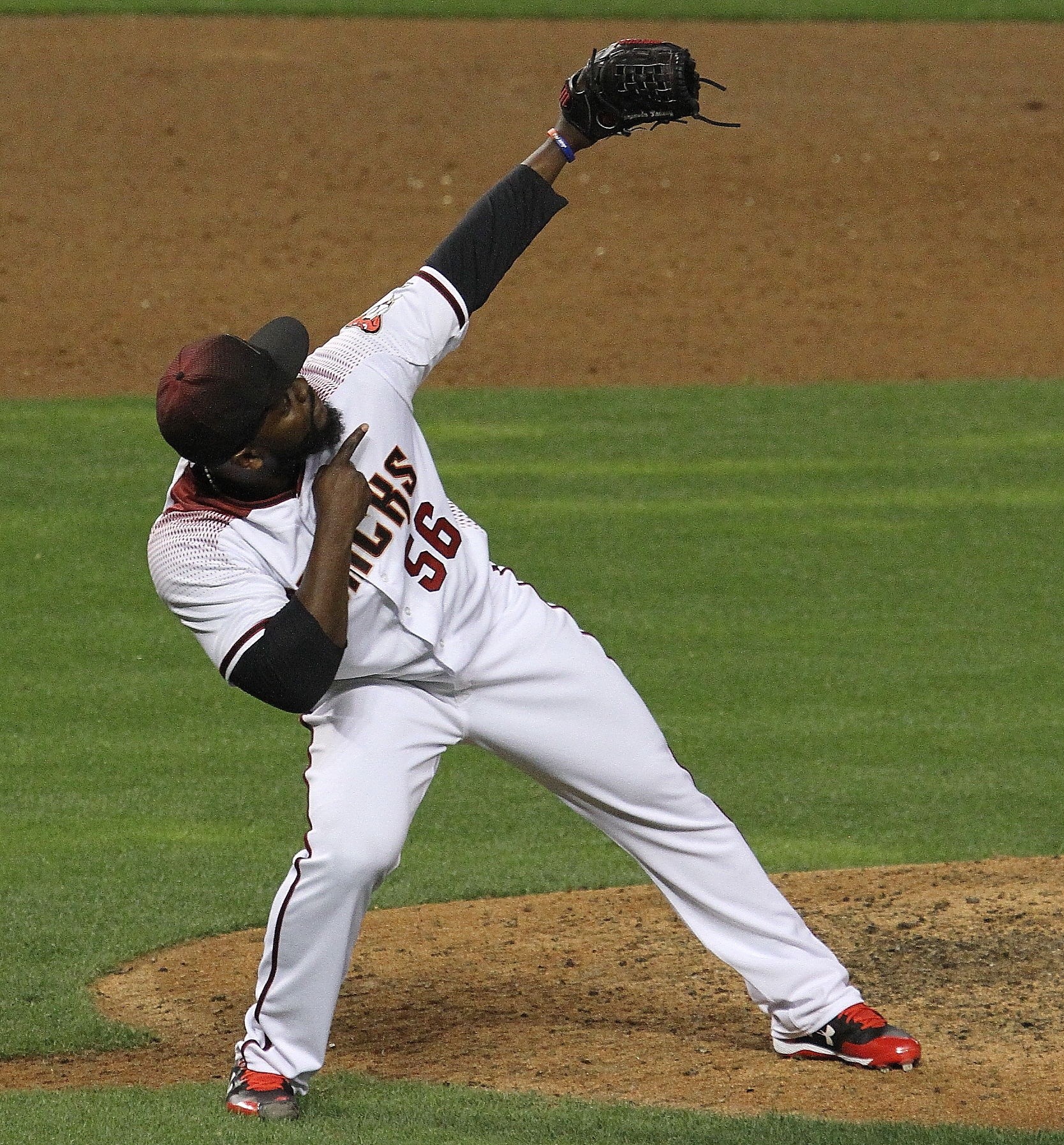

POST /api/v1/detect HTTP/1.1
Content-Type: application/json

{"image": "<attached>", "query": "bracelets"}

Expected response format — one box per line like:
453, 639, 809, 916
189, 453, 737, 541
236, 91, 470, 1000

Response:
546, 124, 575, 165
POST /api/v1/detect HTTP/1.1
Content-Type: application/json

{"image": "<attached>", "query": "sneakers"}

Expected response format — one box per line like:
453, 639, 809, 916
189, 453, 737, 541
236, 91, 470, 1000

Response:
773, 1004, 921, 1068
226, 1068, 299, 1122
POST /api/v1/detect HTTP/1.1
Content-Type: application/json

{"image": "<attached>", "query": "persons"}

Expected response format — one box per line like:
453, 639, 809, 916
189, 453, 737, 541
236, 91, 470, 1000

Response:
146, 38, 922, 1123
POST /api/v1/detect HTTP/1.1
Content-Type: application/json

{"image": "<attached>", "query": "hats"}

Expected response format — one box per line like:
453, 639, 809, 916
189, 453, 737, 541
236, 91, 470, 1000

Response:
157, 317, 309, 466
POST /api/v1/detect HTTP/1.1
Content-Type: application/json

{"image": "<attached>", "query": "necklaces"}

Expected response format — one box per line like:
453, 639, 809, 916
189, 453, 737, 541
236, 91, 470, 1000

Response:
202, 463, 218, 495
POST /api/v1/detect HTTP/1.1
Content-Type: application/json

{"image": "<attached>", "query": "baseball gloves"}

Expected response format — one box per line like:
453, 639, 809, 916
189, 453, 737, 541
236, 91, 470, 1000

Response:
560, 37, 701, 140
